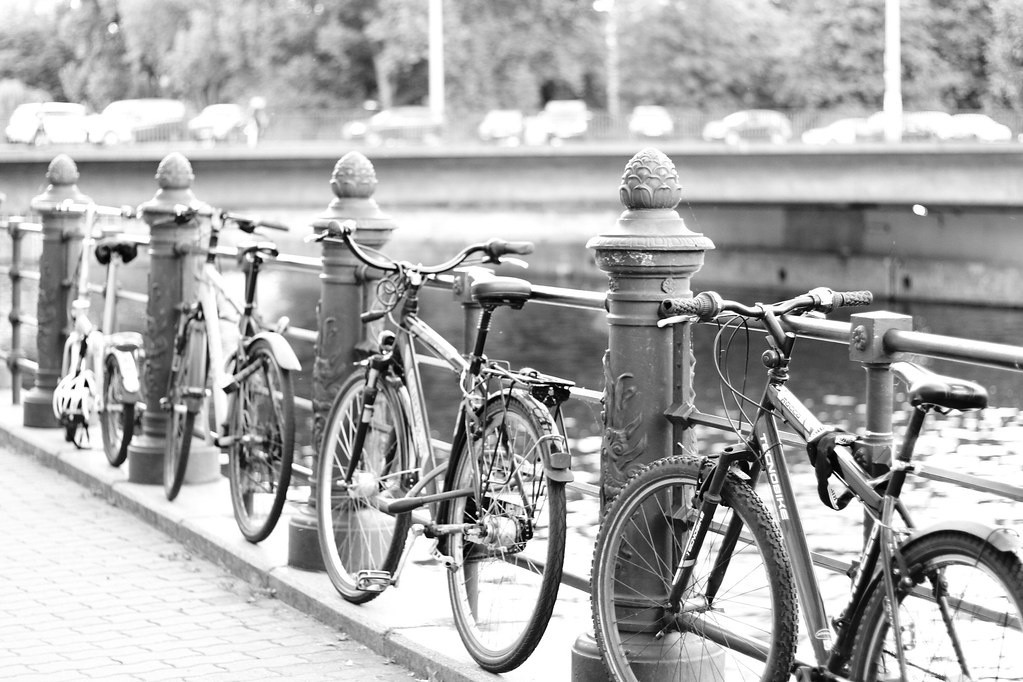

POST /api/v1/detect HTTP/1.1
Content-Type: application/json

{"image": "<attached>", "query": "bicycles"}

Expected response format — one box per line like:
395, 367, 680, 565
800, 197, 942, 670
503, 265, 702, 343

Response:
589, 285, 1022, 682
30, 198, 141, 467
135, 203, 304, 546
304, 217, 578, 674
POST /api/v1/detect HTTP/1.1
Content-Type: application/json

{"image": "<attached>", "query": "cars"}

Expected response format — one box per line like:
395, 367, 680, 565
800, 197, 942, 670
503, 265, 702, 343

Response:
5, 95, 1023, 148
